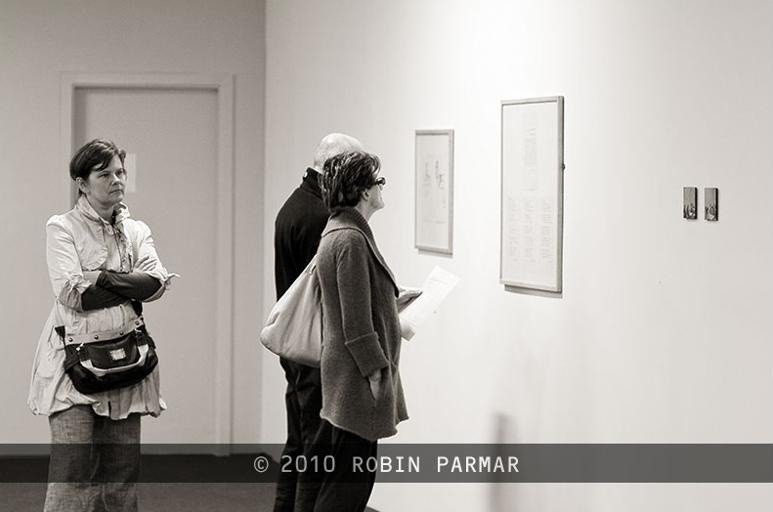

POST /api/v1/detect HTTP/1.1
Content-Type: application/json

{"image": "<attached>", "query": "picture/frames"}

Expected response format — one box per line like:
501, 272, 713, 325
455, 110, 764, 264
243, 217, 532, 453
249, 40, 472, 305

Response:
499, 95, 564, 293
414, 129, 454, 254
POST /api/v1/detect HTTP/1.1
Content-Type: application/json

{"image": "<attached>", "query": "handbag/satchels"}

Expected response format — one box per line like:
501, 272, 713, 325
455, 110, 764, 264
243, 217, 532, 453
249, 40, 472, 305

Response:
259, 253, 322, 369
62, 314, 158, 395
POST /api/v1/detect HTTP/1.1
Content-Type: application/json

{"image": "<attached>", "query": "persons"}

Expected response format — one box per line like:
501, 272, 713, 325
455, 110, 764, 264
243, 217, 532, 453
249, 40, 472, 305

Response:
296, 148, 409, 512
271, 131, 420, 512
27, 136, 181, 512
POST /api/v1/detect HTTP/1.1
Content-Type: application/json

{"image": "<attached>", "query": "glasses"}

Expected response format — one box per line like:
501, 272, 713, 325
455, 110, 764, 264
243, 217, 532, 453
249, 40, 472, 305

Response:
372, 177, 385, 186
